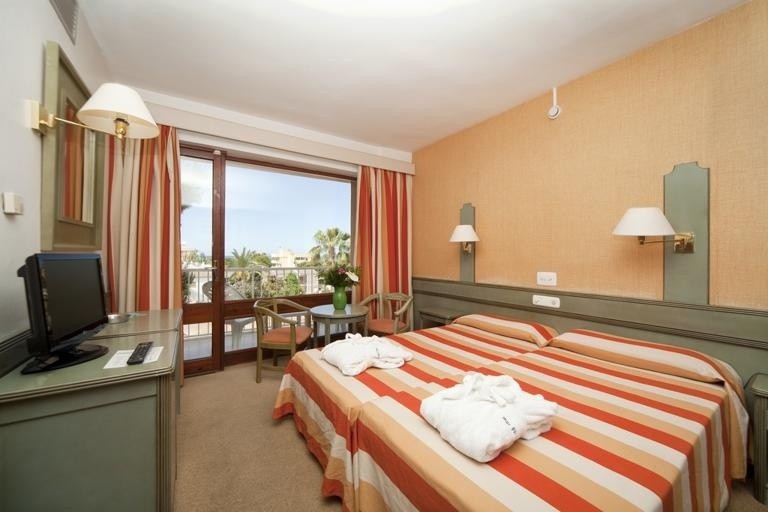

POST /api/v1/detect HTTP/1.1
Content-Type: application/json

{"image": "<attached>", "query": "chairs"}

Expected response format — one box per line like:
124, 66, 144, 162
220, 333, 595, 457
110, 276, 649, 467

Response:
202, 281, 256, 351
253, 299, 315, 384
359, 292, 413, 336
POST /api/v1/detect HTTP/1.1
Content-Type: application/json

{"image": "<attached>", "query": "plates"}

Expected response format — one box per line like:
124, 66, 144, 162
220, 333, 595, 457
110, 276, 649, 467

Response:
108, 314, 130, 324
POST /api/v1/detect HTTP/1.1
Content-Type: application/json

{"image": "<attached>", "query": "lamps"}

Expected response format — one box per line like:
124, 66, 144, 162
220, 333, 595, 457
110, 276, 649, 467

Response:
30, 82, 160, 139
448, 224, 481, 254
612, 207, 695, 253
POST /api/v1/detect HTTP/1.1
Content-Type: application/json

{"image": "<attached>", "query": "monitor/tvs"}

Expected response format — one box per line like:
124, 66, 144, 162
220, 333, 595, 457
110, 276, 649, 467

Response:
17, 253, 109, 375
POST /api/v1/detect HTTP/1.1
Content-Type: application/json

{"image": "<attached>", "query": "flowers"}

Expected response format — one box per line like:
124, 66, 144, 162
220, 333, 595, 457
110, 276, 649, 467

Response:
309, 228, 361, 289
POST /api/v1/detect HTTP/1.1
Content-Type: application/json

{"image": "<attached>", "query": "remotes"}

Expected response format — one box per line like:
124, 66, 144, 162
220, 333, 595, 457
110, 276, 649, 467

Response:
127, 342, 152, 365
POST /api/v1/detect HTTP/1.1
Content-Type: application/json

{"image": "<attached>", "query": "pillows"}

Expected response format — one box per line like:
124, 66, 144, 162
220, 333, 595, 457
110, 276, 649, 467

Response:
541, 328, 723, 384
450, 312, 558, 347
41, 39, 105, 255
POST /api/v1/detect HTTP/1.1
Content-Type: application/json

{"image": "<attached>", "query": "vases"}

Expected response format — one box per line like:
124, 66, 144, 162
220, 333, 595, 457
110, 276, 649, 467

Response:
332, 285, 347, 309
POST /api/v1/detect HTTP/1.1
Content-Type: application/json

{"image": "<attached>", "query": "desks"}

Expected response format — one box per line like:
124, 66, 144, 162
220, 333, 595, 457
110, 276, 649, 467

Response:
1, 330, 183, 510
277, 310, 308, 328
309, 304, 370, 349
87, 308, 184, 415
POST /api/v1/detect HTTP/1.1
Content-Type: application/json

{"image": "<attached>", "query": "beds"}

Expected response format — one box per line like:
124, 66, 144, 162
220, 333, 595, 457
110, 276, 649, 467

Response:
272, 277, 767, 512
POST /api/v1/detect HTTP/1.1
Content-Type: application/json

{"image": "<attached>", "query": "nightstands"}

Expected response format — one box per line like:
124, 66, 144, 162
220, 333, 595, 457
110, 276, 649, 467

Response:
751, 372, 768, 506
